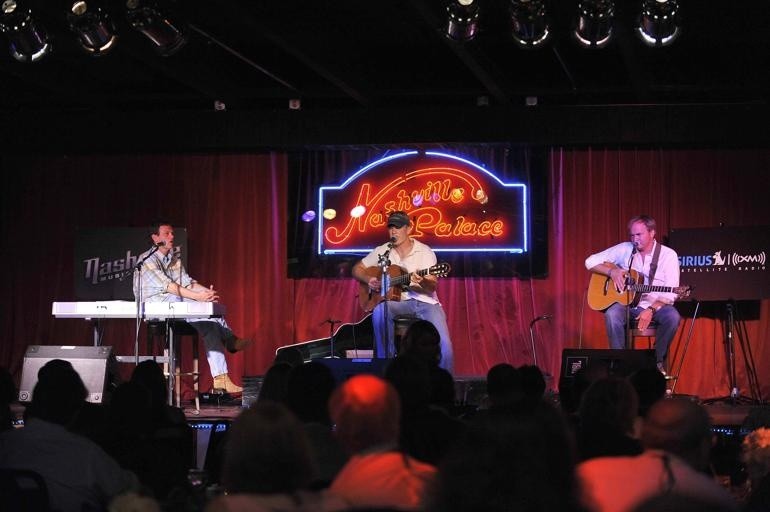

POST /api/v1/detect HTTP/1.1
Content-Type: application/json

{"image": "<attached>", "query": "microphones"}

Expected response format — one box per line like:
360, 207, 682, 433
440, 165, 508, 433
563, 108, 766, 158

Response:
388, 233, 396, 249
152, 240, 166, 247
632, 239, 640, 248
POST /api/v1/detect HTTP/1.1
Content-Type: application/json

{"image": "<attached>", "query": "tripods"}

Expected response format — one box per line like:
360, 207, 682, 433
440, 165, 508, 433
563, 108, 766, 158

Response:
674, 304, 770, 403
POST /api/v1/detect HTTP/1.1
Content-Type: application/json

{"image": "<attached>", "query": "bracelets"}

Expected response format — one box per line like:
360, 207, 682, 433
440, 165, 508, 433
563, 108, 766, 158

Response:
607, 270, 612, 278
648, 306, 656, 315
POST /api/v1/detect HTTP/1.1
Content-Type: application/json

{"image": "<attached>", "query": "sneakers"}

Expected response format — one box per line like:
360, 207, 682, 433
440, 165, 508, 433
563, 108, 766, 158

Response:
659, 363, 678, 380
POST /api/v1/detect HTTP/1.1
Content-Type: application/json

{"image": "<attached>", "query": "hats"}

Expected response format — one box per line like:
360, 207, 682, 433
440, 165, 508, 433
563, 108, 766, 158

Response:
387, 213, 410, 228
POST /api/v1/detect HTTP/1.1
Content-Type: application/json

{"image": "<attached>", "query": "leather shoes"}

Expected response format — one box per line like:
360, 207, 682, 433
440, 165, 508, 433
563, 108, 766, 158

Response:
224, 335, 253, 353
213, 374, 244, 393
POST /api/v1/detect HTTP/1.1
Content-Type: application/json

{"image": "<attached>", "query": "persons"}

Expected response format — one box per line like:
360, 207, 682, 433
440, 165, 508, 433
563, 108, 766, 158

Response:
132, 220, 251, 394
584, 216, 682, 381
351, 211, 453, 373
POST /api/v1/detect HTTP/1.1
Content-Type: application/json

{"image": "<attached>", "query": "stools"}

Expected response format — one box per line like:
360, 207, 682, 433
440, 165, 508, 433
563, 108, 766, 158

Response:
146, 320, 200, 412
623, 318, 670, 392
393, 317, 420, 352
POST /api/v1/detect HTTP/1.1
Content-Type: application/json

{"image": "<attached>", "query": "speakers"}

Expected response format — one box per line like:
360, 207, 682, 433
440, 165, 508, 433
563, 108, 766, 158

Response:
314, 357, 394, 390
18, 343, 119, 404
559, 348, 675, 409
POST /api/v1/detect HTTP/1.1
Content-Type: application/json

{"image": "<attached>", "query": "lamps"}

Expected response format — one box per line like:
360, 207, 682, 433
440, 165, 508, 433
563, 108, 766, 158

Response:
1, 1, 195, 65
441, 0, 684, 51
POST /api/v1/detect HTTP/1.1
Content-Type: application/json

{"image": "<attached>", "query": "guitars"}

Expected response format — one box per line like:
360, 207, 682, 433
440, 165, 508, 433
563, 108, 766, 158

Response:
587, 263, 692, 313
358, 263, 452, 314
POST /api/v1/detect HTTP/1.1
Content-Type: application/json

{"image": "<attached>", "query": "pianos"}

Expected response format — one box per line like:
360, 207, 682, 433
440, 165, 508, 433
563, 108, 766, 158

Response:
51, 299, 228, 318
